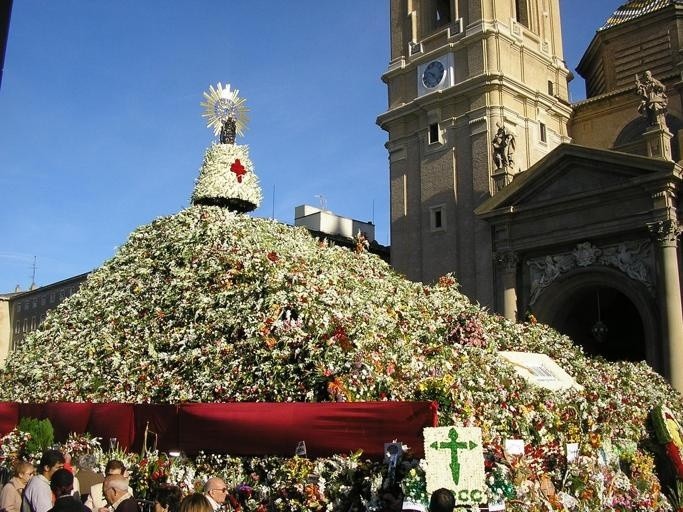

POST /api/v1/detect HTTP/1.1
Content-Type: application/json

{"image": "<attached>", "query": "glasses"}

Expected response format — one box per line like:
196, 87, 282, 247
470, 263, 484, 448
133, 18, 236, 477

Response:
24, 473, 36, 476
213, 488, 226, 493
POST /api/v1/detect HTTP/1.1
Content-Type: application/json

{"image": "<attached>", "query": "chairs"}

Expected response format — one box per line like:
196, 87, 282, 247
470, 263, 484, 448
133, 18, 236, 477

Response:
135, 498, 154, 512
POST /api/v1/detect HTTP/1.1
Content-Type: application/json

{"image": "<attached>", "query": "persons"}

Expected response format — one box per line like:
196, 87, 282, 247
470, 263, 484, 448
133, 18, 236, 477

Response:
634, 70, 667, 112
22, 449, 65, 512
60, 454, 81, 498
202, 477, 229, 512
492, 123, 517, 168
427, 488, 455, 512
0, 463, 34, 512
617, 240, 656, 293
178, 493, 214, 512
84, 460, 133, 512
528, 256, 560, 307
153, 488, 181, 512
98, 474, 141, 512
46, 469, 92, 512
380, 485, 404, 512
